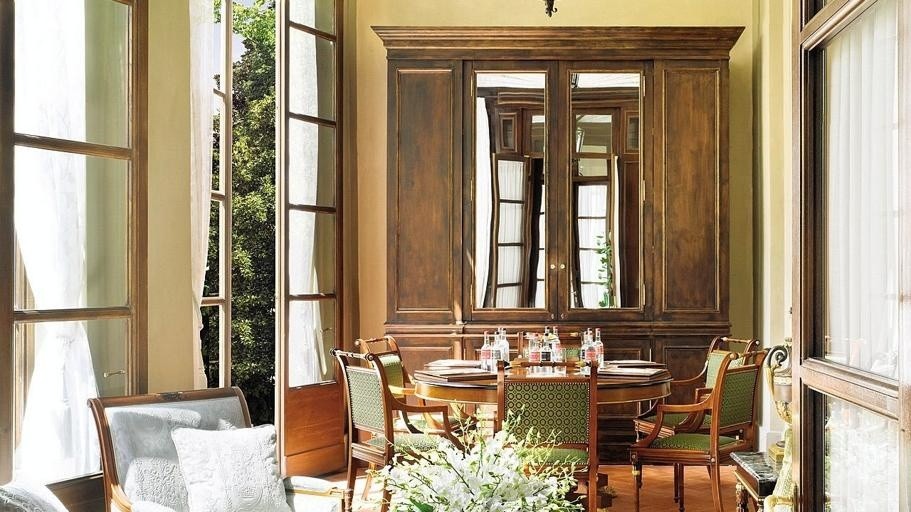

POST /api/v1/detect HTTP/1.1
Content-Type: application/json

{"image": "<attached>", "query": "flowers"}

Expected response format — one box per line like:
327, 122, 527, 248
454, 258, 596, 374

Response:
365, 405, 586, 511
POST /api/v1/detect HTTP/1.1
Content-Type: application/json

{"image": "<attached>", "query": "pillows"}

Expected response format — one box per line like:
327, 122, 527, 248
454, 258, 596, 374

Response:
0, 469, 71, 511
170, 425, 291, 512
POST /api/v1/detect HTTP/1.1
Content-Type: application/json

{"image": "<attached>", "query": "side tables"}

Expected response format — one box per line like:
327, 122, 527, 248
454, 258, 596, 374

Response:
728, 450, 784, 512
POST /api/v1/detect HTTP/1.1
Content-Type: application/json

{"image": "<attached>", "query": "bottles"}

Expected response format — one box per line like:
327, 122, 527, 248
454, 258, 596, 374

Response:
580, 328, 605, 371
480, 326, 510, 372
528, 326, 562, 363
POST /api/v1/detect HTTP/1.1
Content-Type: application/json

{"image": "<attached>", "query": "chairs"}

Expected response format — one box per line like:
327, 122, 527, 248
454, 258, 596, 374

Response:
624, 348, 771, 512
328, 346, 471, 512
353, 336, 480, 441
493, 360, 613, 512
632, 335, 761, 503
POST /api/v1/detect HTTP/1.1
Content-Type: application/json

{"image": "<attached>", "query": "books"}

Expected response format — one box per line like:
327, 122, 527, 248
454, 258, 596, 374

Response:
413, 356, 497, 382
598, 359, 668, 382
